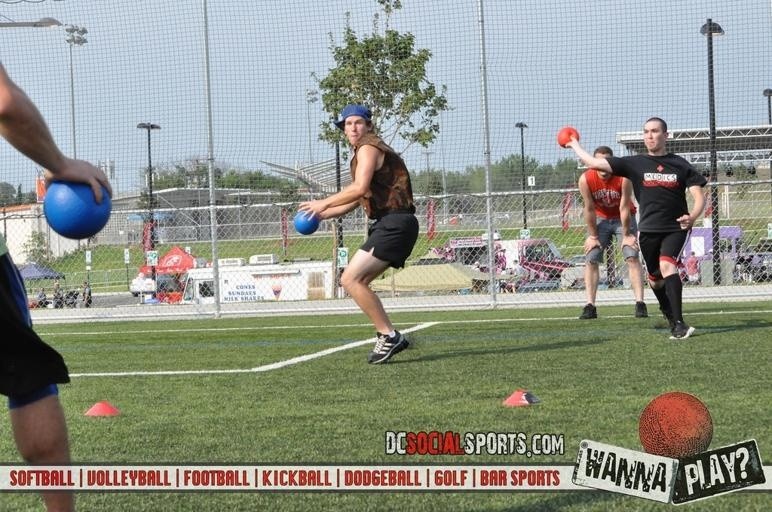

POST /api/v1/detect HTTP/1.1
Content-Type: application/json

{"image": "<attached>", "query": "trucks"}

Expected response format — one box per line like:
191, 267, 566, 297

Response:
180, 262, 345, 305
444, 237, 570, 283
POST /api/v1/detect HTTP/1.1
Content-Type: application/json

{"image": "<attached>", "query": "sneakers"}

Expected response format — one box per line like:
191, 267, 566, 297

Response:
669, 321, 695, 340
579, 303, 597, 319
372, 328, 404, 364
368, 332, 409, 363
635, 301, 648, 317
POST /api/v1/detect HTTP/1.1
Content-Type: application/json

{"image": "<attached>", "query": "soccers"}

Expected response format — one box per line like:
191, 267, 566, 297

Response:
639, 393, 713, 457
294, 212, 318, 235
558, 128, 579, 148
43, 179, 111, 239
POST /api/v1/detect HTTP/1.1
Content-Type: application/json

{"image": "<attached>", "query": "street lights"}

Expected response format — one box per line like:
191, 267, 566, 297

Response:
515, 121, 534, 234
63, 20, 87, 160
137, 119, 163, 297
761, 89, 771, 187
700, 19, 726, 283
421, 151, 436, 194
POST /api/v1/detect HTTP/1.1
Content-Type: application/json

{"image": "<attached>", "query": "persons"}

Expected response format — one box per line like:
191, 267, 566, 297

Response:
38, 286, 48, 308
504, 258, 523, 295
0, 57, 116, 502
575, 143, 652, 322
562, 114, 707, 343
686, 251, 701, 283
52, 282, 65, 307
294, 100, 421, 368
82, 280, 92, 307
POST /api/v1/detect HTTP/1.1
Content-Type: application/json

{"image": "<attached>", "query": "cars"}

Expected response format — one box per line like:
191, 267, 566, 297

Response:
293, 257, 314, 262
570, 256, 610, 284
130, 271, 157, 297
193, 254, 276, 268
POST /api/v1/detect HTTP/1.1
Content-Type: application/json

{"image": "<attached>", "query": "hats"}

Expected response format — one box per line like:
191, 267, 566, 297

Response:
334, 104, 372, 130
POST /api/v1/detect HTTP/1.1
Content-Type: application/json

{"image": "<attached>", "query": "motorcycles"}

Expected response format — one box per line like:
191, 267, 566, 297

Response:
66, 291, 79, 308
